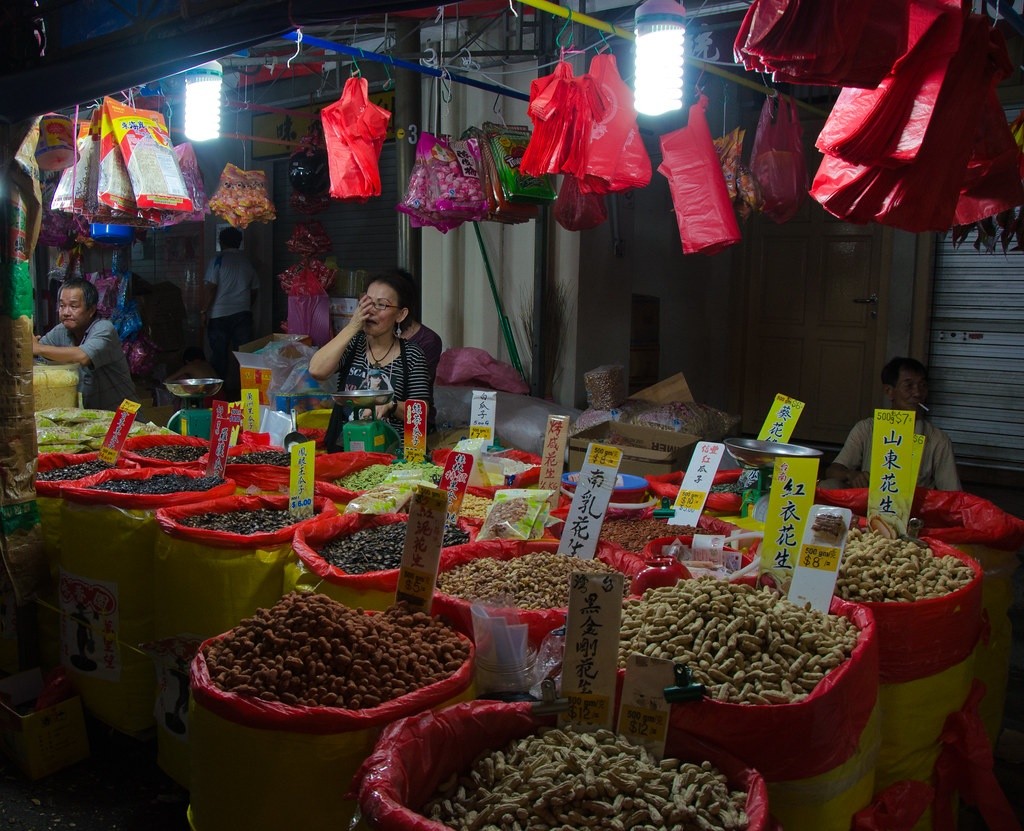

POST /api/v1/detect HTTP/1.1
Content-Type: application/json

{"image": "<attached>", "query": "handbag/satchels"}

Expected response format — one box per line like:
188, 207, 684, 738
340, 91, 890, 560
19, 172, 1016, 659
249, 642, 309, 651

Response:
749, 91, 810, 223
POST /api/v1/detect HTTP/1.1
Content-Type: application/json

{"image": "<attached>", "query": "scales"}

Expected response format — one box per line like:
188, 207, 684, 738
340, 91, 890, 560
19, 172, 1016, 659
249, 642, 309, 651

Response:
723, 437, 823, 523
328, 390, 402, 455
160, 379, 224, 442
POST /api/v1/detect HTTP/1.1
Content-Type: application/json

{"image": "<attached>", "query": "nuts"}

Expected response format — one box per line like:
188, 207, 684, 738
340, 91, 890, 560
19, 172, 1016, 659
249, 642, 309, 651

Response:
30, 443, 976, 831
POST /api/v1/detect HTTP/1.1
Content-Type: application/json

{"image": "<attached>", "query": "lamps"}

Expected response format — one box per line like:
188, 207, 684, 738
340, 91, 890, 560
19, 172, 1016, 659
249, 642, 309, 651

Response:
183, 59, 223, 142
634, 0, 685, 116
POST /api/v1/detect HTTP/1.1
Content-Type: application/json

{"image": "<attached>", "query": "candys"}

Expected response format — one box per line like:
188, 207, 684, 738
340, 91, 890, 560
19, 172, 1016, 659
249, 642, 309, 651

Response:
403, 160, 485, 220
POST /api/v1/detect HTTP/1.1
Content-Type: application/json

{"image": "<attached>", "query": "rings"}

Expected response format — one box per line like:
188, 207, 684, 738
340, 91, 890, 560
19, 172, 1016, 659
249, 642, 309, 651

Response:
359, 304, 361, 307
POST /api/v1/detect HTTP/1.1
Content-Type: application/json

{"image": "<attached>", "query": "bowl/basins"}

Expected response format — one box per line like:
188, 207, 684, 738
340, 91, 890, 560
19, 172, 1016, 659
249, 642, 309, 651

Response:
630, 554, 693, 594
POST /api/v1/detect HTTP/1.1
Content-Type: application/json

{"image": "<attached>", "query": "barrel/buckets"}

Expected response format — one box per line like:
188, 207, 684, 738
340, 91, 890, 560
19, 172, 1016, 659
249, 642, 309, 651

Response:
561, 472, 648, 503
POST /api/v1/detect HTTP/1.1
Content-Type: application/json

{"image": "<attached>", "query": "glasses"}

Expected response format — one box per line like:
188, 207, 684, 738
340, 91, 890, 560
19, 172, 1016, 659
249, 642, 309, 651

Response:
365, 296, 403, 310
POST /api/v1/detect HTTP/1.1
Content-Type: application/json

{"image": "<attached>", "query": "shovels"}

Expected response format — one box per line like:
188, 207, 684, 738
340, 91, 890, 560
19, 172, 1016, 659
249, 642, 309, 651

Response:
283, 408, 309, 453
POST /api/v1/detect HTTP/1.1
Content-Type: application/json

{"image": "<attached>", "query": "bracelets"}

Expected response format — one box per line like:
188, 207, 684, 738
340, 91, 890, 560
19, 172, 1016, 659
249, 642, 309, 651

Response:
199, 311, 205, 314
387, 398, 397, 414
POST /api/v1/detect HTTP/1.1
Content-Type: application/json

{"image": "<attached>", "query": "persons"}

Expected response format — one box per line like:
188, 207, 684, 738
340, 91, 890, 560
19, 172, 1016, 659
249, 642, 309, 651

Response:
307, 274, 429, 455
199, 227, 259, 392
388, 268, 442, 434
165, 346, 225, 409
818, 358, 963, 491
33, 277, 145, 423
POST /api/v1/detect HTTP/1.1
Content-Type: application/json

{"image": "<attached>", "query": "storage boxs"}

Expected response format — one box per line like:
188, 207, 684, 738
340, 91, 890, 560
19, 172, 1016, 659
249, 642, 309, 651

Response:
137, 389, 175, 426
565, 421, 703, 478
232, 333, 313, 406
332, 299, 359, 333
0, 670, 92, 781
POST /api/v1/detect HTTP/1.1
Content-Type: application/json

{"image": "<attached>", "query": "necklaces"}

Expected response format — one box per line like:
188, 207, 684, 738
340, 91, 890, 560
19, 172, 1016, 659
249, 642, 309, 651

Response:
368, 336, 395, 368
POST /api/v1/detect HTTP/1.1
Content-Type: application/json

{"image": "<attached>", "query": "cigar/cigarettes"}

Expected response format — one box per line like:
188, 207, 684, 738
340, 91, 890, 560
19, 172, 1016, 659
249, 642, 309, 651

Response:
918, 403, 929, 411
